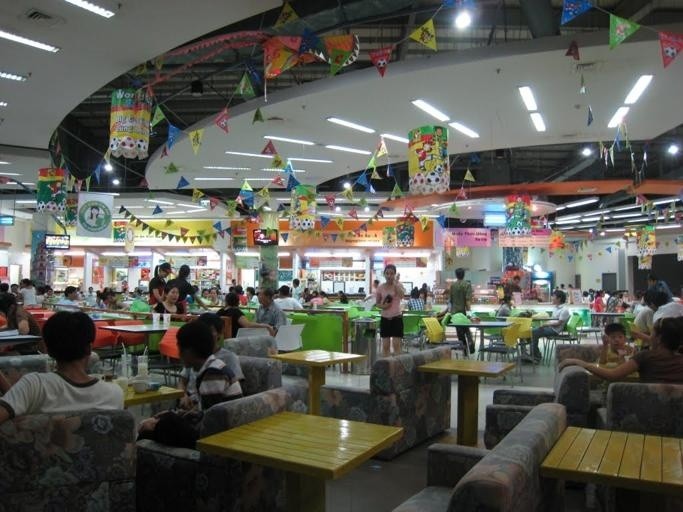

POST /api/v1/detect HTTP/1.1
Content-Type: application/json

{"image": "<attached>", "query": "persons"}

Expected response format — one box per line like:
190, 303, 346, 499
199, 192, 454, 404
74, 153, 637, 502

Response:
523, 290, 569, 365
504, 275, 523, 308
448, 268, 475, 355
0, 262, 380, 357
558, 272, 683, 386
0, 310, 124, 424
494, 294, 529, 360
136, 319, 243, 452
165, 313, 245, 414
408, 283, 432, 313
377, 265, 404, 358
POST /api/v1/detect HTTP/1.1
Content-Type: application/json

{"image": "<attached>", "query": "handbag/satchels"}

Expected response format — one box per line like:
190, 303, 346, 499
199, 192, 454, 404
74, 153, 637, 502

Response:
383, 295, 393, 305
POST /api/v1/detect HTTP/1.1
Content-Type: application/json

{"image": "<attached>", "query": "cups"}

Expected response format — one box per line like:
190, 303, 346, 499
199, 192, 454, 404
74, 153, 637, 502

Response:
162, 314, 171, 328
113, 354, 161, 394
152, 313, 160, 326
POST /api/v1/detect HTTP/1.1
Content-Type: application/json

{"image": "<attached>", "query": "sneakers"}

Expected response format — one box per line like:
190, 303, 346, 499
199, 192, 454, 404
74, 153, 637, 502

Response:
534, 355, 542, 360
527, 355, 539, 364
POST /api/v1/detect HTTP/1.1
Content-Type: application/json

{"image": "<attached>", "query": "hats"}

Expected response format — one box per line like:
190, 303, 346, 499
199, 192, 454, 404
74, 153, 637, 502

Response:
160, 263, 173, 273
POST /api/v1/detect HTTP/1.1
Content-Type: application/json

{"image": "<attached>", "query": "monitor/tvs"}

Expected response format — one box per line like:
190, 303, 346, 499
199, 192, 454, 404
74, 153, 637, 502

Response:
0, 215, 15, 226
44, 234, 70, 249
253, 229, 278, 245
400, 282, 414, 298
332, 281, 345, 296
